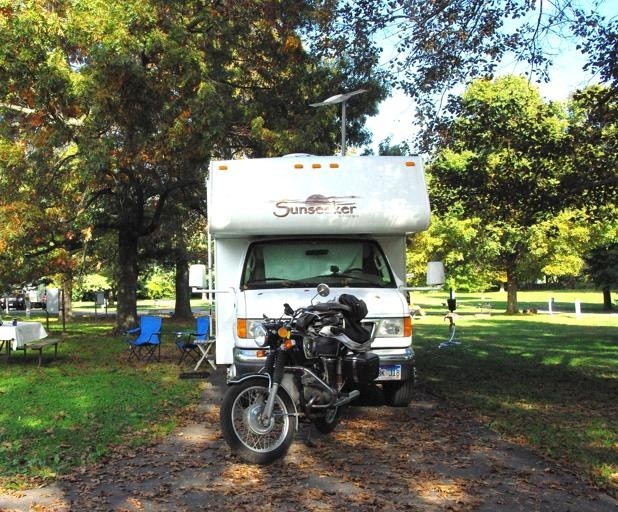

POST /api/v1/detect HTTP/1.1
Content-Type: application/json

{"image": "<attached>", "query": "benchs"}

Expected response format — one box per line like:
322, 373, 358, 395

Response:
32, 337, 61, 369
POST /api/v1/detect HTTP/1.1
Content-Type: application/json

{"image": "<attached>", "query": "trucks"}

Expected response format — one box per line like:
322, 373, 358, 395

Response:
17, 291, 47, 310
189, 151, 446, 407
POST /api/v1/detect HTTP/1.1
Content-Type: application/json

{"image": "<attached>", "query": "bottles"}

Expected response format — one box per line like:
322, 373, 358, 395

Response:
12, 319, 17, 326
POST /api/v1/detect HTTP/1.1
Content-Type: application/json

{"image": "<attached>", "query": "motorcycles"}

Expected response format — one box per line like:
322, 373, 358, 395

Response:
219, 282, 380, 465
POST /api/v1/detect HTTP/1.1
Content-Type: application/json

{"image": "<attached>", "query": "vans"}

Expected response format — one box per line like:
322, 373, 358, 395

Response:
0, 293, 17, 308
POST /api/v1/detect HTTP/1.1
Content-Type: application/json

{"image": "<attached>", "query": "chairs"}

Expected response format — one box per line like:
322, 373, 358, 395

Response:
125, 314, 210, 366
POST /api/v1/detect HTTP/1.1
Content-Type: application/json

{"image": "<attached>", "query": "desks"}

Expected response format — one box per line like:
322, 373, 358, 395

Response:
1, 320, 42, 360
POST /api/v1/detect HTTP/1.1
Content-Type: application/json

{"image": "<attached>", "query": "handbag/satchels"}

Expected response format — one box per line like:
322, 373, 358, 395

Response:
321, 318, 371, 351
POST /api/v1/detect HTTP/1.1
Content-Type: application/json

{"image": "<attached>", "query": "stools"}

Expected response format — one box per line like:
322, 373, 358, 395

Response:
193, 339, 216, 371
